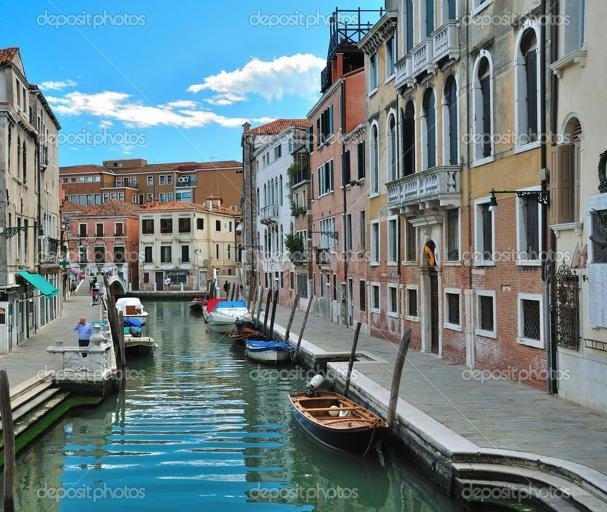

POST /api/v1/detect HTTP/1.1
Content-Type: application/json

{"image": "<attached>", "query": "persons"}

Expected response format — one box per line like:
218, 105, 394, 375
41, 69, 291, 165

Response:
167, 276, 171, 291
73, 316, 94, 357
163, 277, 168, 292
222, 280, 228, 299
239, 284, 243, 300
68, 276, 97, 297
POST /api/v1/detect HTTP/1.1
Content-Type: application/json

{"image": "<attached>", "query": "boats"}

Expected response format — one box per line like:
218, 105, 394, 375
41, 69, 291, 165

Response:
288, 390, 386, 455
115, 297, 158, 354
190, 297, 292, 364
